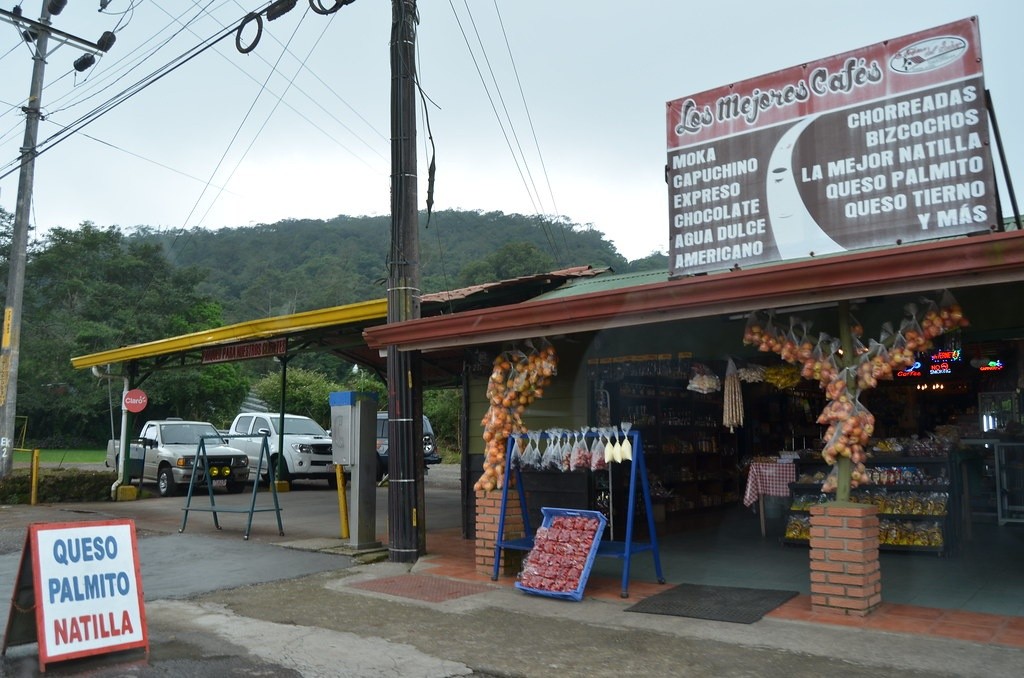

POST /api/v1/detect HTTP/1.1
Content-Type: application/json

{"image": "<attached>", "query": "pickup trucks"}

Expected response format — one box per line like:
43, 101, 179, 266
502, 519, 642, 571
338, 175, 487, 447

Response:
106, 420, 250, 495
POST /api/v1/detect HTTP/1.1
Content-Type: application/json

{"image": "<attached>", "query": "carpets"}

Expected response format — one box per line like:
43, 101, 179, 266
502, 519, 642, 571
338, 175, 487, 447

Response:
624, 583, 800, 625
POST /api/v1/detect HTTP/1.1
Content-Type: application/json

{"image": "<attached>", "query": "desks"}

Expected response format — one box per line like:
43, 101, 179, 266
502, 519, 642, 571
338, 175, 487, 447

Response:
744, 462, 797, 537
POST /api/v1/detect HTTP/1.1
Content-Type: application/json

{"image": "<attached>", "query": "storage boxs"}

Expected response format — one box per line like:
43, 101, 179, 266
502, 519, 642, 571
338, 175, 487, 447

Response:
514, 506, 609, 602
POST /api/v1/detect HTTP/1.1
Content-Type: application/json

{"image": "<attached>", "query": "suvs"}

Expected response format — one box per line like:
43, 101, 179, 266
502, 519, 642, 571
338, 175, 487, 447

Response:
329, 408, 442, 485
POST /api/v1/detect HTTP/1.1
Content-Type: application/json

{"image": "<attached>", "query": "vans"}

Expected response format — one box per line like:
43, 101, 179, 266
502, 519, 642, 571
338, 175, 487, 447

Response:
227, 412, 349, 489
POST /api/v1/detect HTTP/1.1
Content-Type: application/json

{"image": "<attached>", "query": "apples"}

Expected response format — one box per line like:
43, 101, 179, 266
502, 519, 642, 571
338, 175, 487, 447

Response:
474, 347, 557, 491
744, 304, 970, 492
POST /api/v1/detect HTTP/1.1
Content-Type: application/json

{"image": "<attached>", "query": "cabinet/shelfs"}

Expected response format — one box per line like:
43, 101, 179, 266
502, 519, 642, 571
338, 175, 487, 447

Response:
960, 438, 1001, 517
591, 375, 743, 523
995, 442, 1024, 526
749, 393, 828, 455
777, 452, 962, 560
